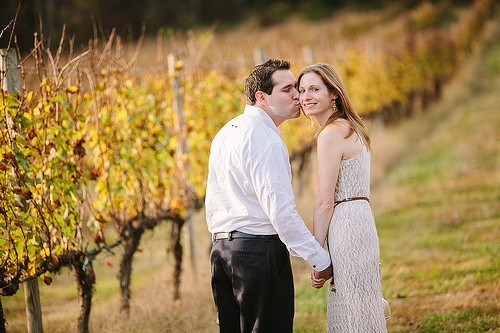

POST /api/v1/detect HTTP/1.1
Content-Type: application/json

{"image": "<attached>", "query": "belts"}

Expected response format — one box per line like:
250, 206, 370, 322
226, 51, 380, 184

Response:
214, 231, 278, 239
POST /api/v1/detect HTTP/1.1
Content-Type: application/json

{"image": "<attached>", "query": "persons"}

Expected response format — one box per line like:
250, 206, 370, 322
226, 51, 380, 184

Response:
204, 59, 334, 333
298, 63, 388, 333
